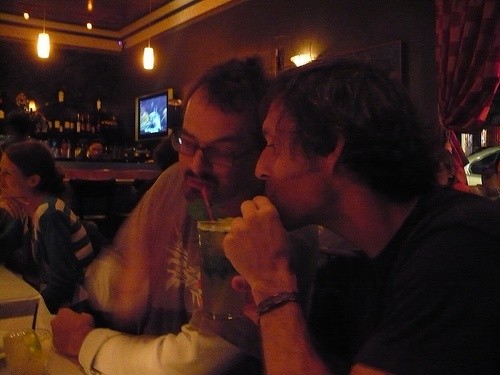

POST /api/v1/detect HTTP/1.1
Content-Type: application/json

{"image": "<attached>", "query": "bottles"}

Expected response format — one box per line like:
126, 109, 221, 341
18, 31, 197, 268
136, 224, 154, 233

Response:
58, 87, 65, 104
0, 98, 5, 119
28, 109, 120, 161
96, 96, 103, 111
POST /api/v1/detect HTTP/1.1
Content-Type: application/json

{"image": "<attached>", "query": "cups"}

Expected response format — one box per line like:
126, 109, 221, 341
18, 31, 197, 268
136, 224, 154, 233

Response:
2, 327, 53, 374
196, 217, 246, 322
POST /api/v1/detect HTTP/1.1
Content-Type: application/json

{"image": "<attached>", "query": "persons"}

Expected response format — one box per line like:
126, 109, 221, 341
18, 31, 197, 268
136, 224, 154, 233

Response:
50, 56, 320, 375
0, 143, 97, 310
0, 110, 35, 144
149, 106, 160, 128
78, 138, 106, 162
222, 55, 499, 375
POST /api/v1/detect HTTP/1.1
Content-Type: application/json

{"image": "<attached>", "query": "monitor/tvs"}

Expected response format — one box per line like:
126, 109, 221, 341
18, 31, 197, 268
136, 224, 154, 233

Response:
134, 88, 173, 143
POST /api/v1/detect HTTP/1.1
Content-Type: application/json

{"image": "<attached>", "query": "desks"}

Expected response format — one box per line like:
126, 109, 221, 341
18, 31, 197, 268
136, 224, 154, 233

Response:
0, 263, 89, 375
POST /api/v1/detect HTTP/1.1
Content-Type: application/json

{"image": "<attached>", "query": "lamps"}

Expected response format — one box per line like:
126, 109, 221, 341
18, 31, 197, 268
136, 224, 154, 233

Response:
37, 0, 50, 58
142, 0, 155, 70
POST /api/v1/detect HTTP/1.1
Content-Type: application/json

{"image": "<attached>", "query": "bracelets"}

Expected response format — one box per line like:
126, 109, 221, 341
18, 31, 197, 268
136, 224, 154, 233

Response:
255, 291, 301, 315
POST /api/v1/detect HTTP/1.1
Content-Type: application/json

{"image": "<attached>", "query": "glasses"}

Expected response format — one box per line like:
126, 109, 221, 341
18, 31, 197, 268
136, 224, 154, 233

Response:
169, 130, 261, 171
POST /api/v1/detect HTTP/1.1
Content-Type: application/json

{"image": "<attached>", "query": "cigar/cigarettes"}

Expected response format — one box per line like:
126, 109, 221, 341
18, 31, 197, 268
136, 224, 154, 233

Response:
197, 218, 234, 232
